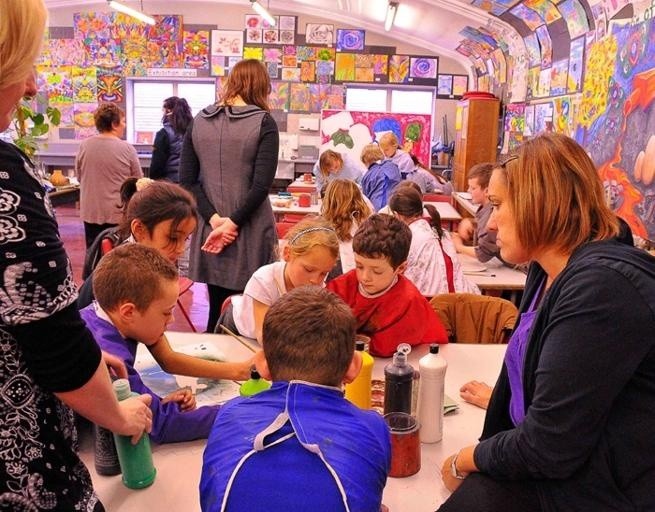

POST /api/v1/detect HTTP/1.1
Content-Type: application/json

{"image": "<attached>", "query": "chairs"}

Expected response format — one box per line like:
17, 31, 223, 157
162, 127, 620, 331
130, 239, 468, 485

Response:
213, 293, 243, 336
101, 237, 197, 334
276, 213, 302, 240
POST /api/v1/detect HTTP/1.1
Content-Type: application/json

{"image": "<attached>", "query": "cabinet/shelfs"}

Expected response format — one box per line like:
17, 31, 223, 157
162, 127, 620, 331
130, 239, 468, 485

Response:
452, 96, 502, 233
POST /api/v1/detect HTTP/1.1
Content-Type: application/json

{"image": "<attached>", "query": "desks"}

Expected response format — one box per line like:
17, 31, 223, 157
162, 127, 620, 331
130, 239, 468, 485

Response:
420, 190, 480, 224
340, 239, 528, 290
77, 329, 510, 511
41, 174, 80, 216
267, 173, 322, 217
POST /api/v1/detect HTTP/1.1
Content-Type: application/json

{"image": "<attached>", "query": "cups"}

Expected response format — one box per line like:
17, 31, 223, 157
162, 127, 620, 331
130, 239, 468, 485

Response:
303, 172, 312, 181
298, 195, 314, 208
271, 195, 293, 209
378, 412, 420, 478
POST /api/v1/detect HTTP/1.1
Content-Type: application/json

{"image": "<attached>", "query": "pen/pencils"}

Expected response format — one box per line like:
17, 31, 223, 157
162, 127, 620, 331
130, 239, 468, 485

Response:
464, 272, 495, 277
215, 323, 256, 354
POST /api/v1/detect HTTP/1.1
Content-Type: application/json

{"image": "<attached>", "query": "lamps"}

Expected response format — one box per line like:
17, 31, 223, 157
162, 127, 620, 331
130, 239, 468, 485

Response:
248, 0, 276, 28
381, 1, 398, 33
105, 0, 155, 28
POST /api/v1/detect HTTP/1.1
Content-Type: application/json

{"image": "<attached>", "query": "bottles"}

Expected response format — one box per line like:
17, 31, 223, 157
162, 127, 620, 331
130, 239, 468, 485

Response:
344, 341, 374, 411
416, 344, 447, 445
240, 368, 270, 395
94, 377, 158, 491
385, 344, 415, 413
